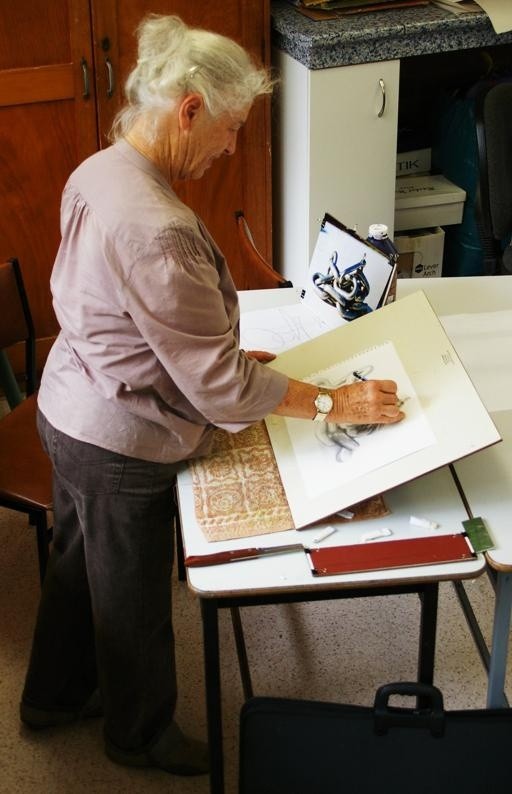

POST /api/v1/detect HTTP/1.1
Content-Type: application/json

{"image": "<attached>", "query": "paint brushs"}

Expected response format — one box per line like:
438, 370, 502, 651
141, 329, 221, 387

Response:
353, 372, 405, 405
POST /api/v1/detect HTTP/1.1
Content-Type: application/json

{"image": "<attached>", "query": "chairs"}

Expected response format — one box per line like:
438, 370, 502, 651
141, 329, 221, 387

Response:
0, 257, 60, 596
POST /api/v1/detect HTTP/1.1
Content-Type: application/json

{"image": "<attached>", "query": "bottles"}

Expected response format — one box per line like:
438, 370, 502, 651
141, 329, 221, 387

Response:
366, 223, 399, 306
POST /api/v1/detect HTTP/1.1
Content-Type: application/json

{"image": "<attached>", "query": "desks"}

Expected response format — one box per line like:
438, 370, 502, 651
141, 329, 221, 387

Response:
381, 272, 512, 710
174, 286, 489, 794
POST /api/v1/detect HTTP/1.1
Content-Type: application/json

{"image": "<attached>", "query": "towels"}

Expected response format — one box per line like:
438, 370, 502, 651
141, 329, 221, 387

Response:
189, 417, 391, 543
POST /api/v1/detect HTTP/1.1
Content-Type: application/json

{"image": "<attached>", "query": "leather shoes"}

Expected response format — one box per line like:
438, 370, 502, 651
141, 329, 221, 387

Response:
20, 692, 104, 727
104, 720, 212, 775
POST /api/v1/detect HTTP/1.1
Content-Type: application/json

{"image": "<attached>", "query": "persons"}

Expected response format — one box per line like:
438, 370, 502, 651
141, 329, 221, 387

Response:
20, 19, 276, 775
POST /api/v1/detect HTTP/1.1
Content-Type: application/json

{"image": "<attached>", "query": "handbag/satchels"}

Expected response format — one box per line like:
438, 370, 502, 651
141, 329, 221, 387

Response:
239, 682, 511, 794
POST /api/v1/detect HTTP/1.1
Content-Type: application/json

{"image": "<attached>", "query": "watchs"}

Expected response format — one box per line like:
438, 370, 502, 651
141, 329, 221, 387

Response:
313, 384, 332, 423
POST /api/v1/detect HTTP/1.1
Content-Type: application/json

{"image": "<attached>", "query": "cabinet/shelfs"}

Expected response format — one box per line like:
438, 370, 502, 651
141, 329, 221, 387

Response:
274, 0, 512, 286
0, 0, 277, 388
